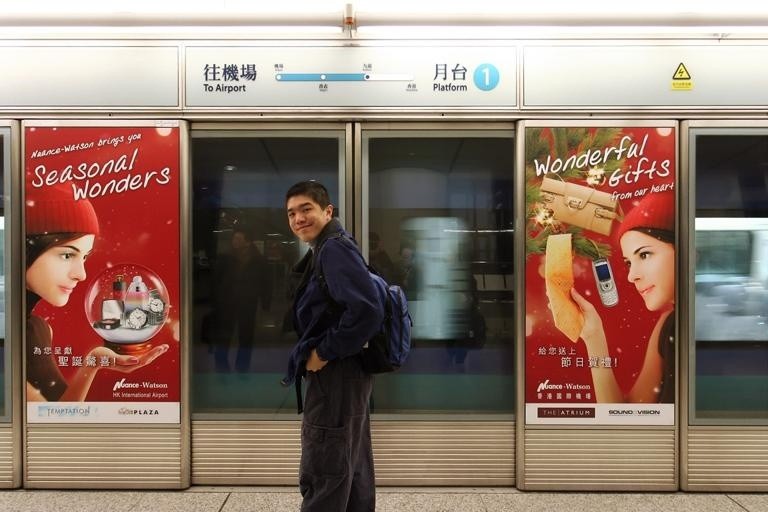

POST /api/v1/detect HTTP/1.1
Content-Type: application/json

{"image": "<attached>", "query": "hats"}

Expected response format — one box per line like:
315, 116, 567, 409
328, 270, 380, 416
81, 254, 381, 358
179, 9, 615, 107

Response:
25, 178, 99, 237
617, 188, 675, 241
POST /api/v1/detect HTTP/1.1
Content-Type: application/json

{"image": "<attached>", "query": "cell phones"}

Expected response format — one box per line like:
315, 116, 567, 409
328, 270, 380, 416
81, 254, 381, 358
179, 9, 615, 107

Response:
590, 257, 620, 308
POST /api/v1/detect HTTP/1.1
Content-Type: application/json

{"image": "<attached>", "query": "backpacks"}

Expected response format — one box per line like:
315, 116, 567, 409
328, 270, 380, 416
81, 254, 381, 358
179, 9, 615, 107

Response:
291, 233, 413, 375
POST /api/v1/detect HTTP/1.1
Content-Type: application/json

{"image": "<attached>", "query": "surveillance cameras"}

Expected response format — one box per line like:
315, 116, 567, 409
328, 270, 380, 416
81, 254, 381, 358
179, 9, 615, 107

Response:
344, 3, 355, 23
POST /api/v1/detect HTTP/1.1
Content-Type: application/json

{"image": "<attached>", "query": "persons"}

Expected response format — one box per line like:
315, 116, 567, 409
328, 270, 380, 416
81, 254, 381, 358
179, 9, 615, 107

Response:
274, 181, 416, 511
199, 231, 271, 384
25, 181, 171, 402
547, 190, 675, 403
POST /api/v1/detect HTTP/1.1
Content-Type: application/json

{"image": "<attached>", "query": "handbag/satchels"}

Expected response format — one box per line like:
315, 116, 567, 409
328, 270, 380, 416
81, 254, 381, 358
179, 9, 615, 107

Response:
192, 312, 217, 353
538, 172, 624, 237
